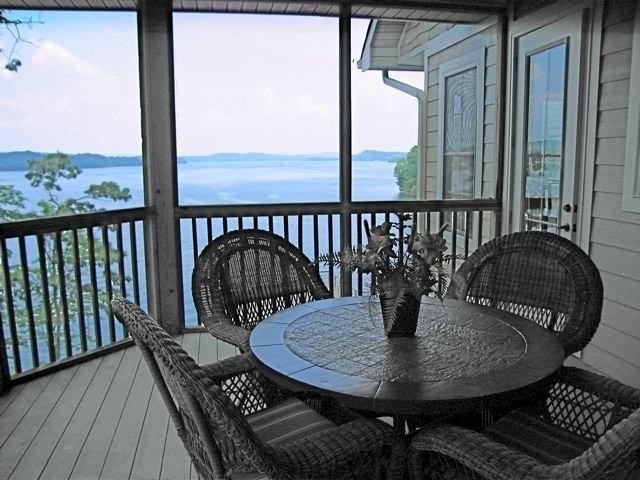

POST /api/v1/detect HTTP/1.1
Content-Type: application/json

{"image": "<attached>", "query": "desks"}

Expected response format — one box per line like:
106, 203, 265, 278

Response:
248, 295, 565, 443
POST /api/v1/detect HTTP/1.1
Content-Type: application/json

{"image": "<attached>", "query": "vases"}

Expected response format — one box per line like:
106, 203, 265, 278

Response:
377, 289, 422, 338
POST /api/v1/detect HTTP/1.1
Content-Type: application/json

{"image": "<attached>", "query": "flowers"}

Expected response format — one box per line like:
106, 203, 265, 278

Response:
306, 206, 486, 338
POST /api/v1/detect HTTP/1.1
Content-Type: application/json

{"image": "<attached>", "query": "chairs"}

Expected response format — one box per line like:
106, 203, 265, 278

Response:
110, 296, 411, 480
190, 228, 333, 357
409, 364, 640, 480
442, 230, 605, 361
